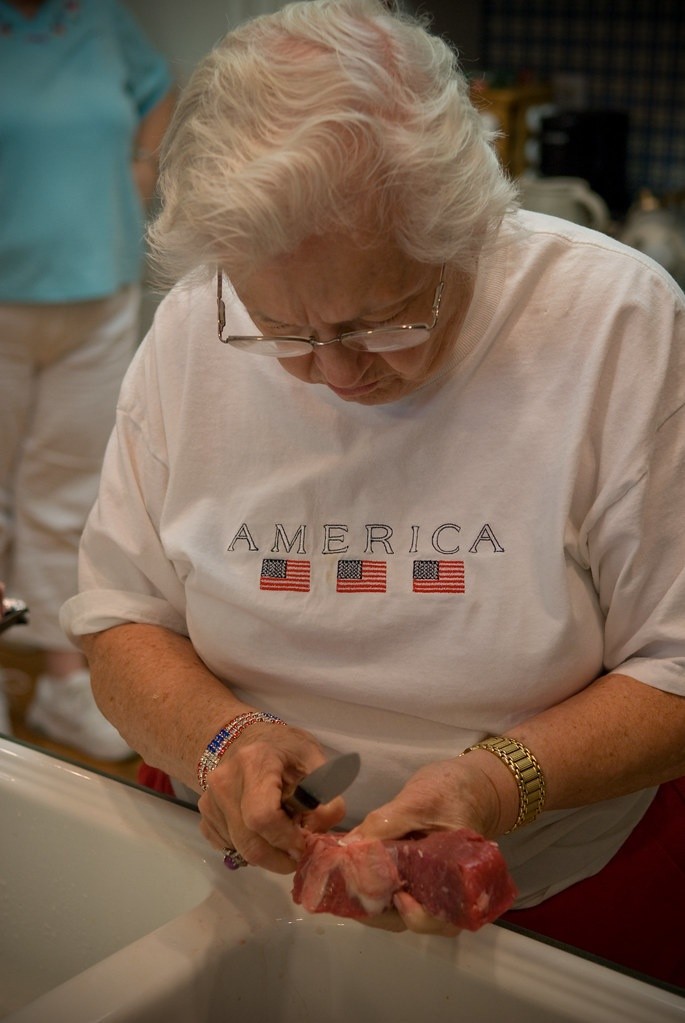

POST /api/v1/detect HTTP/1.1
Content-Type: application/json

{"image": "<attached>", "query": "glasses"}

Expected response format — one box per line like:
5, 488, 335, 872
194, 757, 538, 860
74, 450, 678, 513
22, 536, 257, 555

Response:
216, 264, 445, 358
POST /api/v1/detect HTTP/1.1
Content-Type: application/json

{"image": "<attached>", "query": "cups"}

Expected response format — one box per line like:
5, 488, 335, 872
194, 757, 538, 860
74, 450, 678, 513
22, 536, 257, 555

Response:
517, 177, 609, 231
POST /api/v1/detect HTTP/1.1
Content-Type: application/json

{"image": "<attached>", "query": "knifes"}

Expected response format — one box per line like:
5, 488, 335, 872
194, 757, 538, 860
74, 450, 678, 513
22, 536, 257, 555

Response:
223, 752, 361, 871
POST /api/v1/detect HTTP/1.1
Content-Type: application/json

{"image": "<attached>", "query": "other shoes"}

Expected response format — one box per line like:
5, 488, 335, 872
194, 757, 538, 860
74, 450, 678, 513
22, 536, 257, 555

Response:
27, 668, 139, 762
0, 678, 13, 737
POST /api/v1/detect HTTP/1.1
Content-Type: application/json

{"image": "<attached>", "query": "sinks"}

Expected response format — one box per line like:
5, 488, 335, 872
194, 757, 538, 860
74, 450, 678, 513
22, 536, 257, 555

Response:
0, 734, 262, 1020
11, 882, 685, 1020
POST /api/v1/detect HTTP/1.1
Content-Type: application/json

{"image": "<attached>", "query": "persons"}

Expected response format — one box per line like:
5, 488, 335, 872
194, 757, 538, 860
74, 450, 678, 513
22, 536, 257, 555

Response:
57, 0, 685, 987
0, 0, 178, 764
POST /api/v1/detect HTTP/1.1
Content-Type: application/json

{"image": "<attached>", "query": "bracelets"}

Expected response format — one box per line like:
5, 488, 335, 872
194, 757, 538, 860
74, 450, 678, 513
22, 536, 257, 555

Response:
138, 148, 158, 165
467, 733, 545, 830
199, 713, 283, 791
